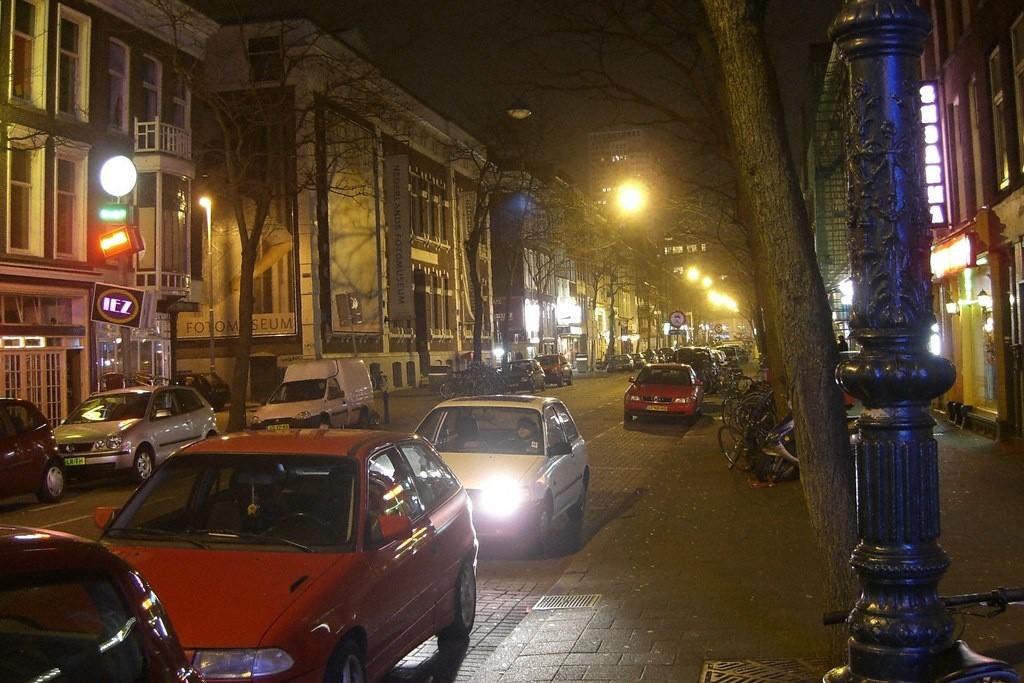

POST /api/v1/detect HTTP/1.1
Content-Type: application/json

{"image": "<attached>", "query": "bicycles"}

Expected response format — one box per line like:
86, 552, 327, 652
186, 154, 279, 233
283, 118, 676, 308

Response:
822, 585, 1024, 683
703, 359, 748, 394
717, 376, 777, 470
439, 362, 507, 398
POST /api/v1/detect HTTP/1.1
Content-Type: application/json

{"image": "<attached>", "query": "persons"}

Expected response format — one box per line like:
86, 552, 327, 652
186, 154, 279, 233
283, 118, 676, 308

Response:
515, 417, 543, 448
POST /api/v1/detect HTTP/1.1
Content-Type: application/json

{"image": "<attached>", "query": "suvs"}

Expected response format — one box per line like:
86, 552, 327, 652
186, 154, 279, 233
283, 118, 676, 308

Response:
175, 372, 230, 411
673, 345, 716, 377
533, 352, 573, 387
659, 347, 674, 360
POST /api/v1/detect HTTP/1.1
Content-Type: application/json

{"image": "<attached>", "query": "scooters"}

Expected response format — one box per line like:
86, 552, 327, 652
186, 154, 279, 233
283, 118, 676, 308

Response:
763, 396, 861, 483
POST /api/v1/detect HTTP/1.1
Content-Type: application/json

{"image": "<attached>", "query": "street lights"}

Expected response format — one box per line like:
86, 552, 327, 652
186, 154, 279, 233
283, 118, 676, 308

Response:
99, 153, 136, 390
198, 195, 216, 373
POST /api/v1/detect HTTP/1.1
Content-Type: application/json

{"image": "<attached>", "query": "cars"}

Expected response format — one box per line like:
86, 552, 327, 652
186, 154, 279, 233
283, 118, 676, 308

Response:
411, 395, 590, 552
710, 344, 749, 362
0, 523, 208, 683
0, 398, 67, 505
606, 354, 635, 373
53, 385, 220, 484
502, 359, 546, 393
641, 351, 659, 364
655, 351, 666, 363
100, 426, 478, 683
624, 362, 703, 422
629, 353, 646, 368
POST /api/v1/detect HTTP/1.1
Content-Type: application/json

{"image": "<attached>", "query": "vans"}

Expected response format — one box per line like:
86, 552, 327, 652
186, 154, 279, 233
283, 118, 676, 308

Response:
251, 358, 374, 432
